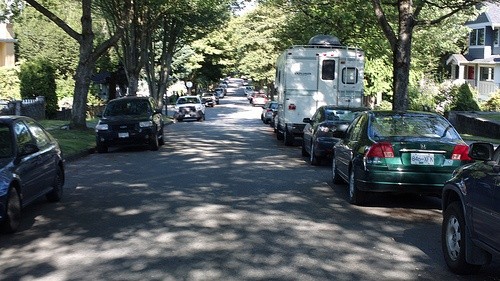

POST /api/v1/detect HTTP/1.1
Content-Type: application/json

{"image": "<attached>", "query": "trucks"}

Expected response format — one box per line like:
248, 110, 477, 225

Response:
275, 34, 366, 148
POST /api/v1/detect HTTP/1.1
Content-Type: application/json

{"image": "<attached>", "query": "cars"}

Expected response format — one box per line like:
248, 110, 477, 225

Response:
243, 86, 277, 122
175, 94, 206, 123
96, 95, 165, 151
332, 109, 465, 205
0, 115, 67, 228
303, 106, 370, 169
440, 142, 500, 281
204, 81, 228, 106
